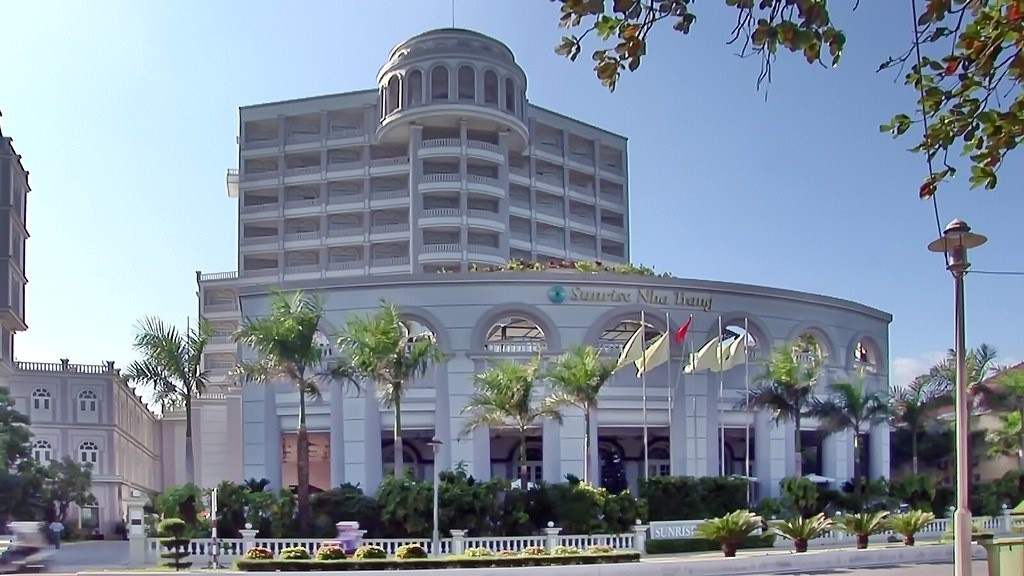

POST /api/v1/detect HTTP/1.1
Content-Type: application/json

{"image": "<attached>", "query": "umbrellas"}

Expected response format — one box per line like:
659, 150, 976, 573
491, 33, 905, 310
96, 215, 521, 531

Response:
803, 473, 836, 515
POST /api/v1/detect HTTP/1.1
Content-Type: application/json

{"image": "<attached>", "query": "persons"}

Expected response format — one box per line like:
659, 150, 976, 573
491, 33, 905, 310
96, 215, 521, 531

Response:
49, 518, 65, 549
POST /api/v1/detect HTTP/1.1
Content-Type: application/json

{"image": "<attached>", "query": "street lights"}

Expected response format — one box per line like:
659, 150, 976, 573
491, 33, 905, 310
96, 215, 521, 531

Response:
925, 218, 990, 576
425, 435, 447, 556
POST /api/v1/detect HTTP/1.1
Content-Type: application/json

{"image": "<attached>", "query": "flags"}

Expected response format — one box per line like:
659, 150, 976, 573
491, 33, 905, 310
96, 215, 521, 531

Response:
610, 318, 744, 378
